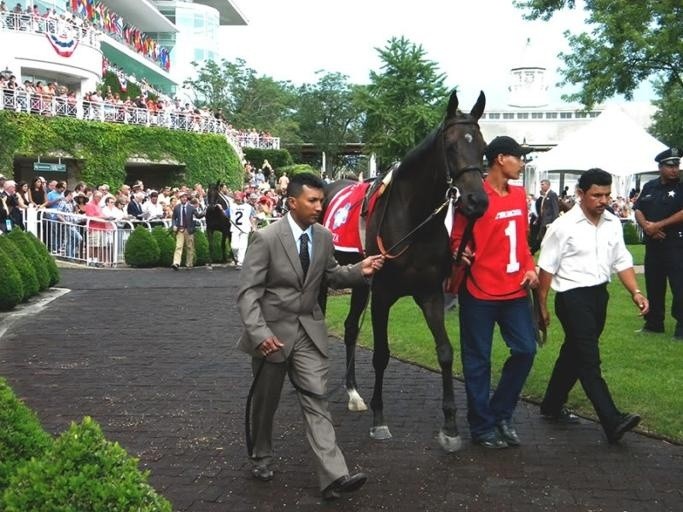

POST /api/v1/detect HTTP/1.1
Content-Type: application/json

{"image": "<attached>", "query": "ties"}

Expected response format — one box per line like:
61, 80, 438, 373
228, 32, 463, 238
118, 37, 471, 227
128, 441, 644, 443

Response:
299, 233, 311, 282
182, 204, 186, 229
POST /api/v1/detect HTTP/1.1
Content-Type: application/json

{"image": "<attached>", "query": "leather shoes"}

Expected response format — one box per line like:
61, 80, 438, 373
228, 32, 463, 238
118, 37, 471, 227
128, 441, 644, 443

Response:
497, 416, 521, 446
322, 472, 369, 502
478, 429, 509, 451
250, 462, 277, 483
633, 320, 666, 336
539, 408, 581, 423
671, 322, 683, 341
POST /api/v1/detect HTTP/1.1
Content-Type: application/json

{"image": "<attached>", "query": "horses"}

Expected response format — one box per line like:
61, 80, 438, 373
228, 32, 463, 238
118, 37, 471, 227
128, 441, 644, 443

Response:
205, 179, 233, 263
317, 89, 490, 454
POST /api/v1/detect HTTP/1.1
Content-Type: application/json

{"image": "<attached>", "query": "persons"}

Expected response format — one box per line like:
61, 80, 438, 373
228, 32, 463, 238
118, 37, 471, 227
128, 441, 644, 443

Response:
0, 75, 227, 134
449, 136, 539, 450
0, 174, 172, 264
241, 159, 291, 225
526, 193, 539, 246
170, 186, 180, 218
180, 185, 194, 201
78, 14, 104, 46
58, 14, 77, 31
535, 191, 546, 228
0, 0, 58, 35
538, 169, 651, 442
170, 193, 207, 272
223, 118, 274, 149
322, 172, 336, 185
557, 185, 576, 216
216, 191, 256, 271
632, 149, 683, 340
220, 183, 234, 199
191, 182, 208, 232
235, 173, 385, 501
606, 188, 642, 228
530, 179, 560, 255
112, 62, 168, 97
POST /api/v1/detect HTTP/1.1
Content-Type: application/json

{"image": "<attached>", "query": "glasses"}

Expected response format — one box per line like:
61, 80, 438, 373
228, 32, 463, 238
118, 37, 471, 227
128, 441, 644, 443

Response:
119, 202, 125, 205
109, 201, 116, 204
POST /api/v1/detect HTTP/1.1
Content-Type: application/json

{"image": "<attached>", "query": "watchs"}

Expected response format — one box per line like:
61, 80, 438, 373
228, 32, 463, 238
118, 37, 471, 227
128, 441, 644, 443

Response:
630, 289, 641, 299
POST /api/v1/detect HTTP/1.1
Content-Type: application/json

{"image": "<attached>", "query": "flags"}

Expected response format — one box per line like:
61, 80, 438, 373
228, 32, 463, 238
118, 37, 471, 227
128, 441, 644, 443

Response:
64, 0, 111, 33
110, 11, 170, 69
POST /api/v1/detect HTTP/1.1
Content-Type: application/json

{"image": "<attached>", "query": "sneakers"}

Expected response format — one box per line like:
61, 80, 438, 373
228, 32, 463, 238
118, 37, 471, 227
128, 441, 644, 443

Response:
236, 261, 243, 267
606, 412, 642, 443
170, 263, 180, 273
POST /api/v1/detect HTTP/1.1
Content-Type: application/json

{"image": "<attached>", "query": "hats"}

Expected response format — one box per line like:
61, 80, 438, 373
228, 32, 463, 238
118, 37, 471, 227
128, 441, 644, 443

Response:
234, 189, 243, 200
39, 176, 48, 183
0, 173, 7, 180
9, 179, 18, 185
132, 179, 143, 189
74, 194, 90, 203
247, 193, 259, 199
178, 191, 189, 198
485, 135, 536, 159
654, 147, 681, 166
